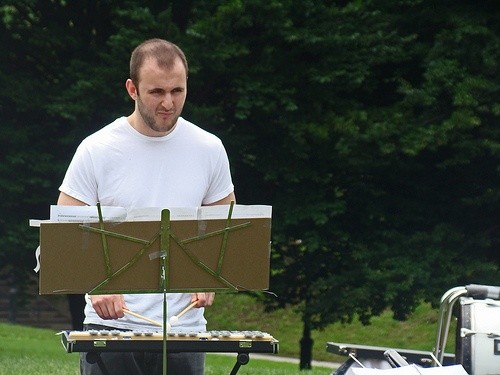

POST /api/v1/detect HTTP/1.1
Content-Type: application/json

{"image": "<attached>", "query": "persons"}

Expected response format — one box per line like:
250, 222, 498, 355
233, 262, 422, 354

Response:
55, 38, 237, 375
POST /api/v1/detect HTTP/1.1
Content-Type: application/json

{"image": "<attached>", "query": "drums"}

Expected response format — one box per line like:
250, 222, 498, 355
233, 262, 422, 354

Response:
455, 297, 500, 375
331, 355, 455, 375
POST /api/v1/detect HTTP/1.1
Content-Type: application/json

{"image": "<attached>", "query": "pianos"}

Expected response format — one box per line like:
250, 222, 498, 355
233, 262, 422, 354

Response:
56, 329, 280, 355
